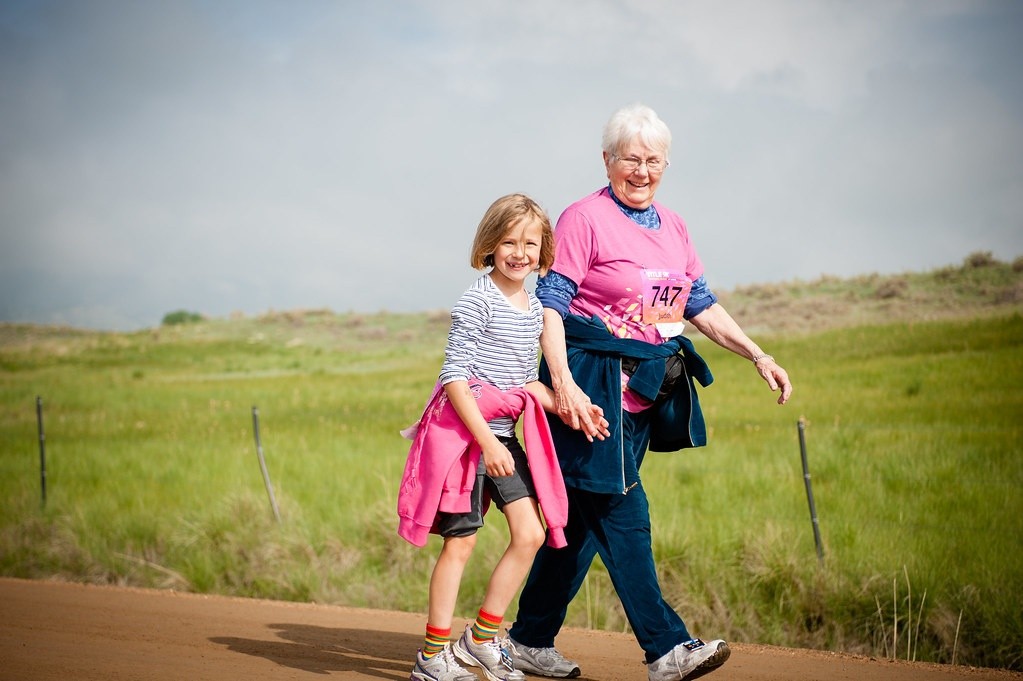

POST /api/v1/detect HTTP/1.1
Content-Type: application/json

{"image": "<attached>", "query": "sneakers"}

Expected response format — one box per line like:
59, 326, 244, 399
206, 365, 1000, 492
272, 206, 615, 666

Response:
452, 623, 525, 681
501, 628, 581, 679
642, 637, 732, 681
410, 641, 479, 681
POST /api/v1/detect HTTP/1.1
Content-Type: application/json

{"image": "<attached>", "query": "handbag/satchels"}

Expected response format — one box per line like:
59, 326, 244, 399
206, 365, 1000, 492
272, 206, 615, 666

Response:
621, 355, 683, 401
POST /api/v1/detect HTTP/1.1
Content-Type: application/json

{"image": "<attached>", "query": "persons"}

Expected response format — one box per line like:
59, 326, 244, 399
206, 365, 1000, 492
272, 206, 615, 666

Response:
504, 107, 793, 681
397, 193, 611, 681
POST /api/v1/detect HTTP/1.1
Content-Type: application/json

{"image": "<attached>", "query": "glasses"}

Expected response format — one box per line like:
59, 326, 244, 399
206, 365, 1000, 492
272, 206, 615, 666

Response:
611, 152, 669, 173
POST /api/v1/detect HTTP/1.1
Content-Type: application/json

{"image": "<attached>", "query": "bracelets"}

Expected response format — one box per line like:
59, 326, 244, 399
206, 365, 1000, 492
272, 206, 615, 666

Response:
753, 354, 776, 365
